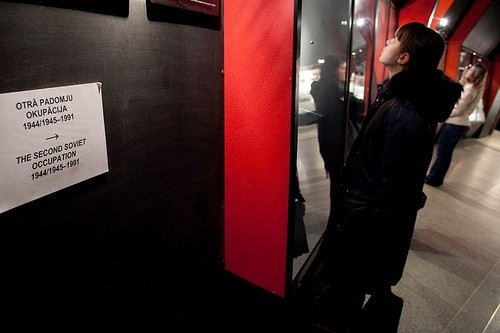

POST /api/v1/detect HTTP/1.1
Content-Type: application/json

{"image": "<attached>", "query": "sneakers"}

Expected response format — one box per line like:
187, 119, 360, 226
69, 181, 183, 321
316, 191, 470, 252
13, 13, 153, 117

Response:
423, 176, 440, 188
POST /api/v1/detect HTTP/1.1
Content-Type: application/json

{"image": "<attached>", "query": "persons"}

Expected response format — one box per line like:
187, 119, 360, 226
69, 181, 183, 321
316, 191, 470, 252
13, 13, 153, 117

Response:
422, 63, 486, 186
308, 21, 463, 333
310, 52, 365, 205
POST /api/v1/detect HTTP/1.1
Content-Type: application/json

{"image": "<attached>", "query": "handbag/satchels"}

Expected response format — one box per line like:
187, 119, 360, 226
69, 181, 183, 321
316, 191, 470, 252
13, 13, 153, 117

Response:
466, 99, 485, 137
